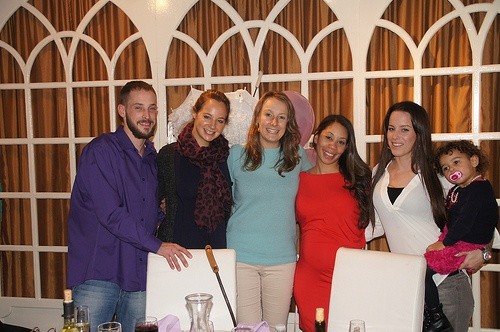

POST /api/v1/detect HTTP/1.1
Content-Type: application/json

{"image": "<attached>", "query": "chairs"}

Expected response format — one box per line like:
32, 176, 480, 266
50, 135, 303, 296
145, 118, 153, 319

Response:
144, 249, 238, 332
327, 246, 427, 332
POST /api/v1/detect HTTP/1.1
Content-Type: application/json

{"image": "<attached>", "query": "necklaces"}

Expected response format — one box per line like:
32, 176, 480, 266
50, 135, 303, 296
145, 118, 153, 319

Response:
450, 174, 481, 203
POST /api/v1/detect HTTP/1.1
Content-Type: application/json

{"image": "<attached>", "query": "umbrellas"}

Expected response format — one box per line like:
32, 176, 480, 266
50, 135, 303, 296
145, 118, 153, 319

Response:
168, 71, 264, 146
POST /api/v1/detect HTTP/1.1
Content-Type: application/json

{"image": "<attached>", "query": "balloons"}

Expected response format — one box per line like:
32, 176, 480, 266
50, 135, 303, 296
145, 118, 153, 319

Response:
278, 90, 317, 166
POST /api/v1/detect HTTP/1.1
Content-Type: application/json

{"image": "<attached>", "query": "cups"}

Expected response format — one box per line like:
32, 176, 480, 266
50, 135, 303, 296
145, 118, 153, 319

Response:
209, 320, 215, 332
135, 316, 159, 332
98, 322, 122, 332
350, 320, 366, 332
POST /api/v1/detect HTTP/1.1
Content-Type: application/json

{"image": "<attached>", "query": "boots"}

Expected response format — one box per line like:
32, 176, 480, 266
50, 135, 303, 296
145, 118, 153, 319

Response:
422, 303, 454, 332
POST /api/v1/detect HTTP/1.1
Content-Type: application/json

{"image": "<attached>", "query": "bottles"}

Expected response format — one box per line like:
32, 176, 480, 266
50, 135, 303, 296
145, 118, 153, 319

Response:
185, 293, 214, 332
315, 308, 326, 332
60, 289, 80, 332
75, 305, 89, 332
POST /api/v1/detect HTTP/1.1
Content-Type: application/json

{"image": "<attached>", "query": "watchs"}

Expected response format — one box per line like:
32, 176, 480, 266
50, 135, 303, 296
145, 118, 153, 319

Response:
479, 247, 493, 263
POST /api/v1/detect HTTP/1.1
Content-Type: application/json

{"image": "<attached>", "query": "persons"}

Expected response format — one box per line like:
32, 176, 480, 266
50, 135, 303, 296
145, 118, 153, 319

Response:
66, 81, 192, 332
365, 101, 494, 332
158, 88, 234, 332
159, 90, 315, 332
295, 115, 371, 332
423, 140, 498, 332
